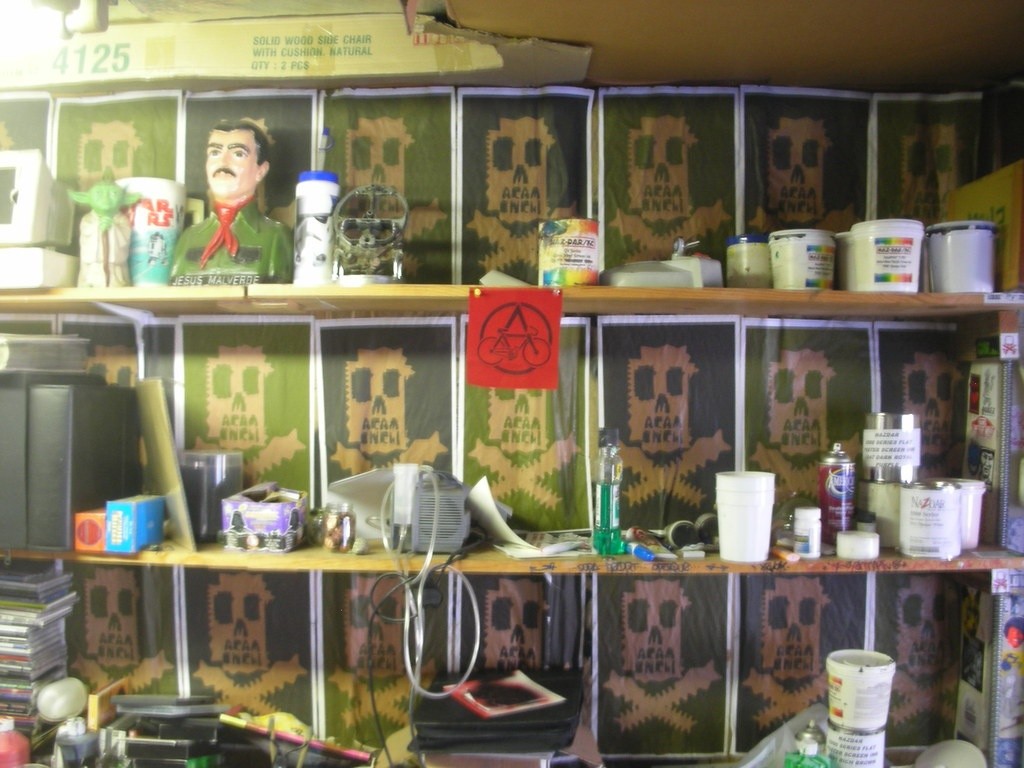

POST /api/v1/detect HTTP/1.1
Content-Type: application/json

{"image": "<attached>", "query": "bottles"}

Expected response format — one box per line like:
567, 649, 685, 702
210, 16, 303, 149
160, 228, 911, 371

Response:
293, 172, 341, 284
592, 427, 623, 556
793, 506, 821, 557
783, 721, 830, 768
858, 509, 877, 536
816, 442, 857, 546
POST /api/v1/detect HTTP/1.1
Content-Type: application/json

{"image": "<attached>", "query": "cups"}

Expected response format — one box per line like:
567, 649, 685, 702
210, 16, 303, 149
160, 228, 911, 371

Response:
717, 471, 774, 562
116, 178, 184, 283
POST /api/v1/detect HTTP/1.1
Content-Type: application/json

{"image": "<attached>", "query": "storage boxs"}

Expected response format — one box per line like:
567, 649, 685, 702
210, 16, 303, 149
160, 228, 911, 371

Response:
74, 506, 105, 555
27, 383, 140, 552
0, 372, 107, 548
422, 722, 604, 768
106, 494, 166, 555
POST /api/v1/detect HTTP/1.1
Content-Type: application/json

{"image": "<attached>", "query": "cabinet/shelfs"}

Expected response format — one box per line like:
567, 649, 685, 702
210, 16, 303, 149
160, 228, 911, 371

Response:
0, 283, 1022, 768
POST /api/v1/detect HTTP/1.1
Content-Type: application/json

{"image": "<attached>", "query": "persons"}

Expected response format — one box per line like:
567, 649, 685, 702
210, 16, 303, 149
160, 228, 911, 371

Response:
167, 120, 294, 285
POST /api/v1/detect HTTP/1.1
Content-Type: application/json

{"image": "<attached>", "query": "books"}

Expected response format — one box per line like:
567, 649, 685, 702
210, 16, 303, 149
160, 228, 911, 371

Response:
0, 558, 80, 753
